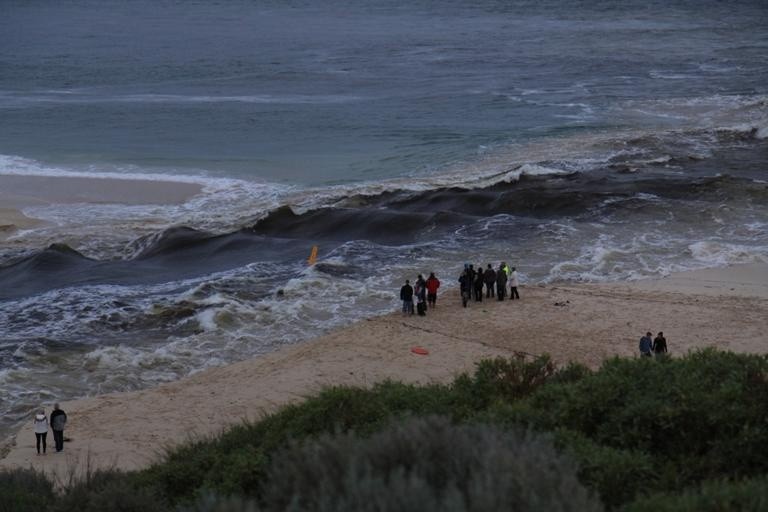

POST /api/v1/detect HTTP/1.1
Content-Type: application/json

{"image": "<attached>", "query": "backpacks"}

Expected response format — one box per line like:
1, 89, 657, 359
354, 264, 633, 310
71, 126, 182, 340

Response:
639, 336, 648, 352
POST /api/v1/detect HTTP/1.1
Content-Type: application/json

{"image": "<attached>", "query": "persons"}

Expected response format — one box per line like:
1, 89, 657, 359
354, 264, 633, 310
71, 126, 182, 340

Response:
640, 332, 653, 355
414, 282, 425, 316
414, 275, 425, 302
425, 273, 439, 302
653, 332, 667, 353
401, 280, 413, 313
34, 410, 47, 453
50, 404, 66, 451
458, 262, 519, 307
